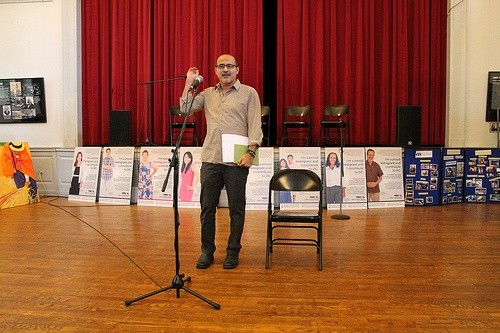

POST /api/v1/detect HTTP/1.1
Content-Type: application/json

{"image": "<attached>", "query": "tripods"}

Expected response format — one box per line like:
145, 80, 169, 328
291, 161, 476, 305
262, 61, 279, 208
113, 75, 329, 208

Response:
125, 87, 221, 311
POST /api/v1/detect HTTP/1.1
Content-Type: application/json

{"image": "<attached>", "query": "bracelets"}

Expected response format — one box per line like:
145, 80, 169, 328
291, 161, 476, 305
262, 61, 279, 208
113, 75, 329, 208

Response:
247, 150, 256, 158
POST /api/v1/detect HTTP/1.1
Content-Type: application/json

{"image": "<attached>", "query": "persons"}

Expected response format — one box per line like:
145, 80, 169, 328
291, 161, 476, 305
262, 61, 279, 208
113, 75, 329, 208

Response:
138, 150, 158, 198
69, 152, 83, 195
279, 159, 292, 203
366, 149, 383, 202
103, 148, 115, 180
179, 152, 199, 202
287, 155, 297, 202
325, 152, 347, 204
180, 54, 264, 269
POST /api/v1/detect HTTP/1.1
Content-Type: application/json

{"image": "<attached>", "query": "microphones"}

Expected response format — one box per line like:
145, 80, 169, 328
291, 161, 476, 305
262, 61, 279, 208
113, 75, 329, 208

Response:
188, 75, 204, 92
339, 118, 343, 125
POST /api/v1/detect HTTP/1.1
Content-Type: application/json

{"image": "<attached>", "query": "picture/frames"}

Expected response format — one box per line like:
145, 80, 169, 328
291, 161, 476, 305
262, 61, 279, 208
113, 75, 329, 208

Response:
0, 78, 46, 123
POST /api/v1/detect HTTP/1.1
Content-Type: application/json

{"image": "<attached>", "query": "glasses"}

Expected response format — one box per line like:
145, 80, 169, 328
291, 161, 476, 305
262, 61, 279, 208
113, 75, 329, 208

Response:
215, 64, 237, 69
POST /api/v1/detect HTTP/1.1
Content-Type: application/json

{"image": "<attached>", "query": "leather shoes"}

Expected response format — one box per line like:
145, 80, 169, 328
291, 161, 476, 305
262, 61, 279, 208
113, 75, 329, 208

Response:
223, 252, 238, 269
196, 253, 214, 269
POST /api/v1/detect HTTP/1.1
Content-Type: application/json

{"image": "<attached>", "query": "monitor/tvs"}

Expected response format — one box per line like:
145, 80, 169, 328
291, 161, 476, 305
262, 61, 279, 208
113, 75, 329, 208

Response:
0, 78, 46, 124
485, 71, 500, 122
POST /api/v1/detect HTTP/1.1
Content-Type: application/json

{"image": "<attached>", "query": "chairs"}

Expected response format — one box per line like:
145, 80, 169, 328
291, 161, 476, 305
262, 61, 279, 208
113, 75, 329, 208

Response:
170, 106, 199, 147
266, 169, 323, 270
261, 106, 271, 146
318, 106, 350, 146
280, 107, 312, 147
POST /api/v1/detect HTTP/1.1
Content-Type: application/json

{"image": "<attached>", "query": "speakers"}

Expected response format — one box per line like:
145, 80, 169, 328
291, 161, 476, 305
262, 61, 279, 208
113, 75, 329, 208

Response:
396, 105, 422, 146
110, 111, 131, 146
